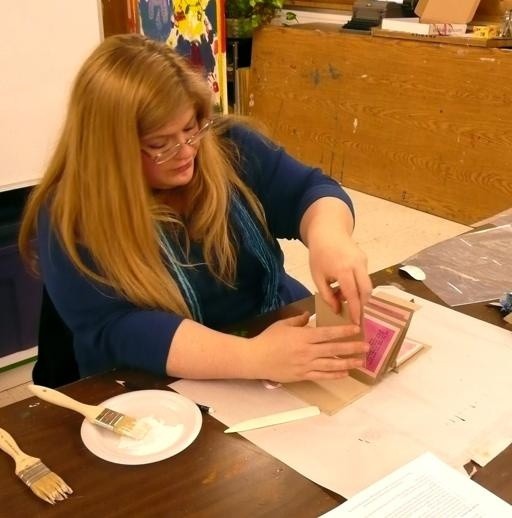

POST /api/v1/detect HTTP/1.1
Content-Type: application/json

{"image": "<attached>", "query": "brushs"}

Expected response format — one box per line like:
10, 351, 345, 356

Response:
0, 427, 74, 506
26, 384, 151, 440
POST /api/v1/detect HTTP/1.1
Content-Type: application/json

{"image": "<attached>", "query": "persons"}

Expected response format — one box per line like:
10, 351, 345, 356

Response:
18, 32, 373, 391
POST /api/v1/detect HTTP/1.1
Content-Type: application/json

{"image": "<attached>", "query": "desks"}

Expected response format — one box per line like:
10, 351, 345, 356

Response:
249, 23, 512, 228
0, 225, 512, 518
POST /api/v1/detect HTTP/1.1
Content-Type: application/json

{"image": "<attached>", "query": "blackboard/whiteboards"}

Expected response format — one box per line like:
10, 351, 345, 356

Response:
0, 0, 105, 192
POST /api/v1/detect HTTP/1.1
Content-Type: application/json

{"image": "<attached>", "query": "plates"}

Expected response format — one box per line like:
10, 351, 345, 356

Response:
79, 388, 202, 467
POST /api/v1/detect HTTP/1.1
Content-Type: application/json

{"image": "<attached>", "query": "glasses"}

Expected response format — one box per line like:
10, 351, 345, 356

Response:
141, 113, 215, 166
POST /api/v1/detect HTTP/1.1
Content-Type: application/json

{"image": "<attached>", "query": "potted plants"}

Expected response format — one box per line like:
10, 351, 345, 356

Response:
226, 0, 296, 39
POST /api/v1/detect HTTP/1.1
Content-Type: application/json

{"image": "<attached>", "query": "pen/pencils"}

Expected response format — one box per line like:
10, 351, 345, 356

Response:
114, 379, 214, 414
